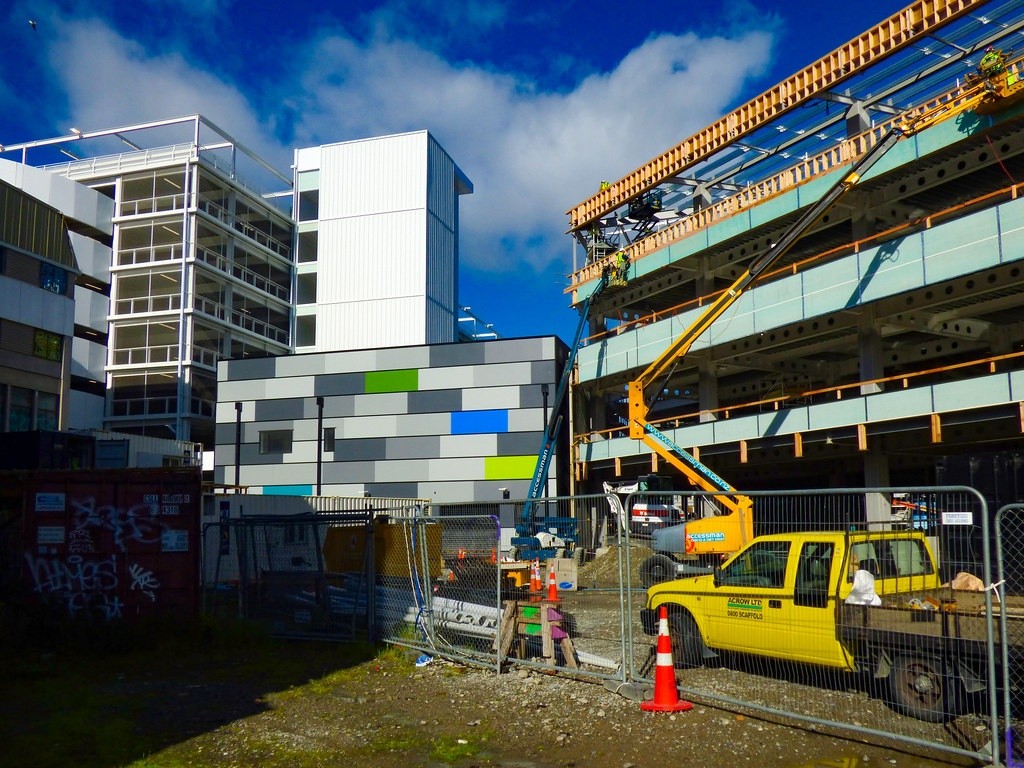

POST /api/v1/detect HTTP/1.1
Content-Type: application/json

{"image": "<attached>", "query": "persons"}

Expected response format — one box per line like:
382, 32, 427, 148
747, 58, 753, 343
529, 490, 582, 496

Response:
599, 180, 610, 191
616, 248, 629, 280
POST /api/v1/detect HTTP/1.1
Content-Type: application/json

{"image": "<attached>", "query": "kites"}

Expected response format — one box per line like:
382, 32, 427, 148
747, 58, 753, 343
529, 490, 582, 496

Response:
28, 18, 39, 32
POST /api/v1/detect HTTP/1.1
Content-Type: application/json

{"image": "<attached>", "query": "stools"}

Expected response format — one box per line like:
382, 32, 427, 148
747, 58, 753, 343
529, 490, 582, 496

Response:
491, 600, 578, 675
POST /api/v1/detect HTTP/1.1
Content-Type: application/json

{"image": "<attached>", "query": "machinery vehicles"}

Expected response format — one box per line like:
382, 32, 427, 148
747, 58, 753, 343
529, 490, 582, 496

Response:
508, 280, 603, 566
625, 68, 1024, 586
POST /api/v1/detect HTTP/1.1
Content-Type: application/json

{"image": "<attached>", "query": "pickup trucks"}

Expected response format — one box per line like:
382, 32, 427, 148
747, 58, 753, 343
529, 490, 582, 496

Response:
641, 529, 1023, 722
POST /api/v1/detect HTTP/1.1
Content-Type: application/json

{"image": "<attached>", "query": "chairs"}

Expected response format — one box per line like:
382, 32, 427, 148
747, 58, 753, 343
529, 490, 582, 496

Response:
796, 557, 829, 595
859, 559, 878, 577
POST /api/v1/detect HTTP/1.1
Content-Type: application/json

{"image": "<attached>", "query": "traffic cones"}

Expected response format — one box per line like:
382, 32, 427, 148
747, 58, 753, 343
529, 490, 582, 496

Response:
489, 547, 499, 565
546, 561, 561, 603
535, 556, 543, 590
457, 545, 467, 561
527, 560, 542, 601
638, 607, 695, 711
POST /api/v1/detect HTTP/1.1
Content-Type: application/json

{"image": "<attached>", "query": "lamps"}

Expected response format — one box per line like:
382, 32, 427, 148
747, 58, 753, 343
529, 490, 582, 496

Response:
498, 487, 509, 494
